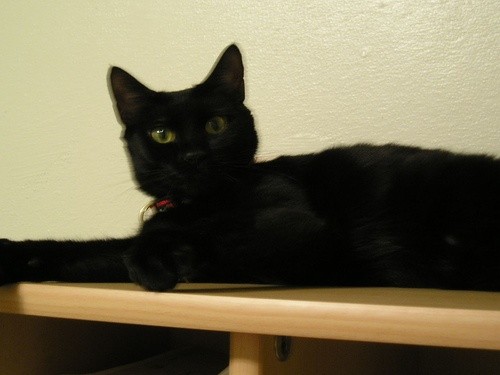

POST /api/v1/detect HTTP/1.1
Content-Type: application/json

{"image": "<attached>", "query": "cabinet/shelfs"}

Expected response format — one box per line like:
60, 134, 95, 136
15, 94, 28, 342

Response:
0, 282, 500, 375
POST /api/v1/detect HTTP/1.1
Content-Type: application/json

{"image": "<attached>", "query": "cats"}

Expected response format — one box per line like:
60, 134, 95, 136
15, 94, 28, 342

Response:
106, 42, 500, 292
